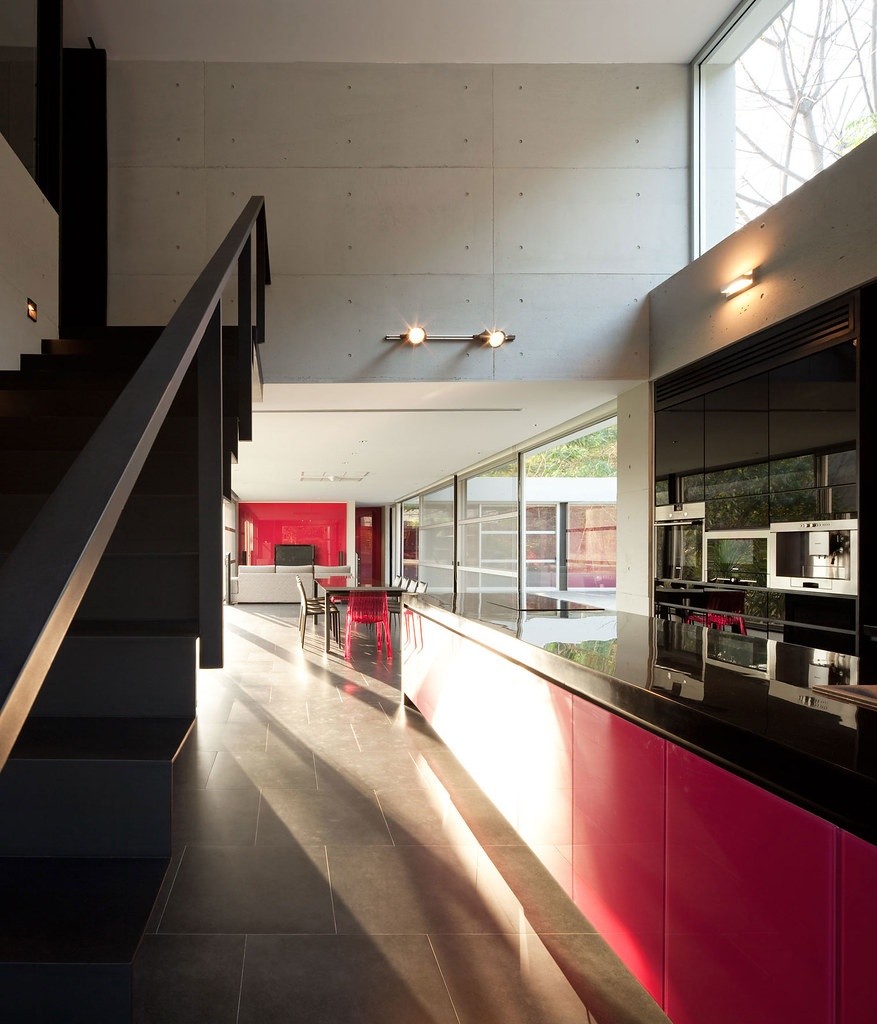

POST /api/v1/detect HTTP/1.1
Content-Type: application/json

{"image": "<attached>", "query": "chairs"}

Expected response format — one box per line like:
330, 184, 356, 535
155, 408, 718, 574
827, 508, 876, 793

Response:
296, 575, 428, 661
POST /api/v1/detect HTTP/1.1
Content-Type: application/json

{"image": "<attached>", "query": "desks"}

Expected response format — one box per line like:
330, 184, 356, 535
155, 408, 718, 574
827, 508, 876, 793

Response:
313, 578, 408, 653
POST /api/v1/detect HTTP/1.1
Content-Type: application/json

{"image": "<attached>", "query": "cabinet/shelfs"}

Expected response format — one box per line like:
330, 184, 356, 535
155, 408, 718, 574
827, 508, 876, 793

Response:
654, 288, 877, 657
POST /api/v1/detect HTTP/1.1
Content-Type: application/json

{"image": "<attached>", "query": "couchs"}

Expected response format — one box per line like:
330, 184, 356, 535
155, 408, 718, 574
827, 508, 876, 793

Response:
231, 565, 351, 604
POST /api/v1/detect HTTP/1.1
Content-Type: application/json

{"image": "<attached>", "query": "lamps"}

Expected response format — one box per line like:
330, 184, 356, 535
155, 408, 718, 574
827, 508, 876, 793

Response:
721, 268, 759, 299
400, 327, 426, 346
474, 329, 506, 348
